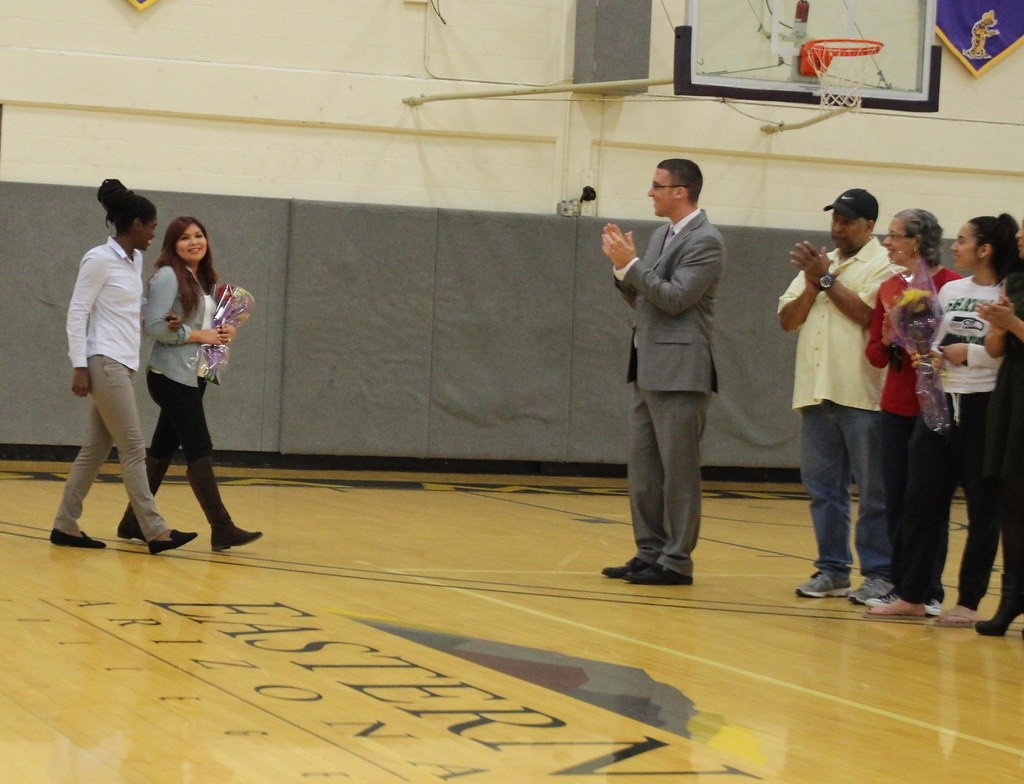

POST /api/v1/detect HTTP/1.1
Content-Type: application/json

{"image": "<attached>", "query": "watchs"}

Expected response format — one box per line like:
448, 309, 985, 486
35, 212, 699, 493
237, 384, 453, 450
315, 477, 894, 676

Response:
818, 274, 835, 291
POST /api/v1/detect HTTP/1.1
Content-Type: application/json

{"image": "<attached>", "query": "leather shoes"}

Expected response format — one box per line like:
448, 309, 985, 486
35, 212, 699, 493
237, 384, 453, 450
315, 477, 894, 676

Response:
622, 563, 692, 585
601, 556, 649, 578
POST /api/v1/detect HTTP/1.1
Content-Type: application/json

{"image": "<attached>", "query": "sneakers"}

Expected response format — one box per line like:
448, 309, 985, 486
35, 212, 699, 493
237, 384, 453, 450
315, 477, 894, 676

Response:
925, 599, 942, 616
847, 576, 894, 606
865, 592, 899, 610
796, 570, 850, 597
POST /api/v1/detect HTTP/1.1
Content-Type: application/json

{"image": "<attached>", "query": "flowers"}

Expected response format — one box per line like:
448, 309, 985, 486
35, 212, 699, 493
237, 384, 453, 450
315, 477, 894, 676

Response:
197, 282, 256, 382
882, 284, 954, 435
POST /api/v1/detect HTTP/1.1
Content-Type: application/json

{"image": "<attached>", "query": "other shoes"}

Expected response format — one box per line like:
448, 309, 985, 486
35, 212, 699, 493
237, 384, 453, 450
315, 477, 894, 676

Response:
148, 529, 198, 555
50, 529, 106, 548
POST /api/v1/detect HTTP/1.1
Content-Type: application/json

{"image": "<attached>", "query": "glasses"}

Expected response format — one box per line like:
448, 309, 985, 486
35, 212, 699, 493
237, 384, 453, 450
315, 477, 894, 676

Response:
653, 182, 688, 190
886, 233, 914, 241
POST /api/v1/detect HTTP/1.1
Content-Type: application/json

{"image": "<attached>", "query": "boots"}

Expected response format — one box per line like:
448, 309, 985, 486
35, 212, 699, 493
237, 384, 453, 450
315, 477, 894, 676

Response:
185, 459, 261, 551
974, 573, 1024, 636
118, 463, 168, 539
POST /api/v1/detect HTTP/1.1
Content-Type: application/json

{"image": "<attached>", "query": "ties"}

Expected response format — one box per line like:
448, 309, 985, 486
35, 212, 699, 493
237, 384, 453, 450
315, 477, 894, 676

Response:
660, 227, 674, 254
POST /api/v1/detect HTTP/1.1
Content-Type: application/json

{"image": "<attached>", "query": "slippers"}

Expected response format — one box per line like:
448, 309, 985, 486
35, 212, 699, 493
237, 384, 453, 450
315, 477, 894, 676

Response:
932, 609, 976, 628
863, 600, 925, 624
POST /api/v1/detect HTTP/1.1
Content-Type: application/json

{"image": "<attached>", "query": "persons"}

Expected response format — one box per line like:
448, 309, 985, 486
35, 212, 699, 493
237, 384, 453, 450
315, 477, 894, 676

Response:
116, 214, 263, 551
601, 158, 728, 585
975, 219, 1024, 636
863, 212, 1019, 627
776, 187, 908, 606
864, 207, 966, 615
49, 178, 199, 555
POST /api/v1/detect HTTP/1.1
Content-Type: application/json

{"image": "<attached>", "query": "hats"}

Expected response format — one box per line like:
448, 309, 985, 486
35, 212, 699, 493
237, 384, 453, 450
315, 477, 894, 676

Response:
823, 189, 879, 221
